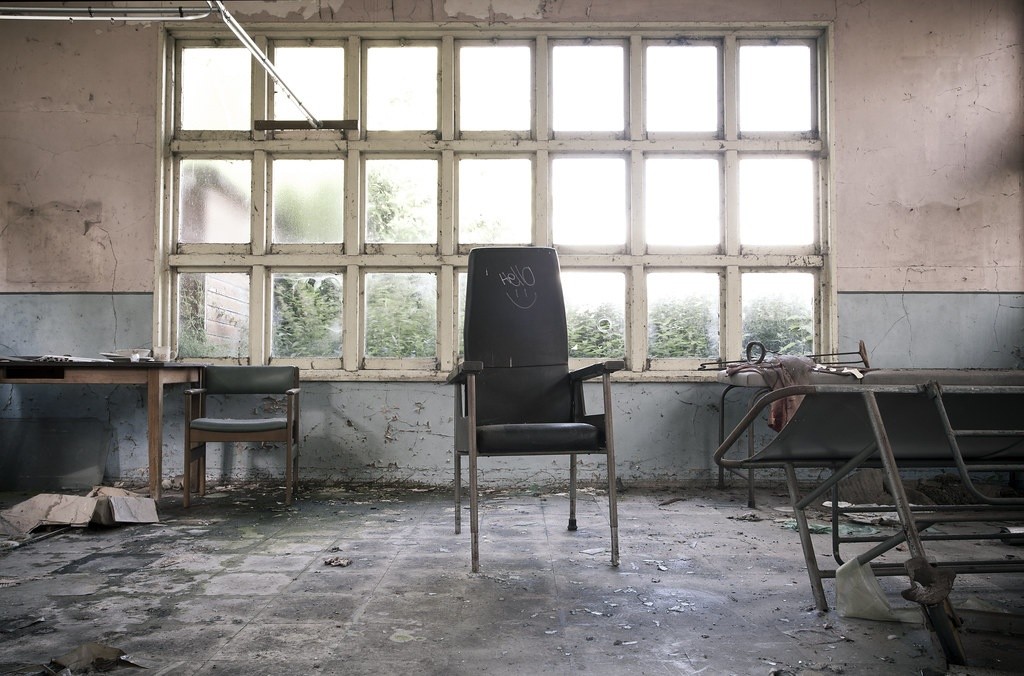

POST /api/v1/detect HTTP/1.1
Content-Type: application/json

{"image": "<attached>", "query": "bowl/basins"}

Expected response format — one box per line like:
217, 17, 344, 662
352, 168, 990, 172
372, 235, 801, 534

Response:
115, 349, 151, 359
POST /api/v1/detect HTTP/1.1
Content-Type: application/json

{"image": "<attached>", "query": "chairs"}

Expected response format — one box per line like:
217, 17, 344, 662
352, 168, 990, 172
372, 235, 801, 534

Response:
185, 366, 301, 506
447, 247, 626, 568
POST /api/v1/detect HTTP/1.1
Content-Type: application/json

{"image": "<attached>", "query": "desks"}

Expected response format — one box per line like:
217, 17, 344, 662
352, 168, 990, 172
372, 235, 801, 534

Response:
0, 359, 208, 503
714, 370, 1024, 507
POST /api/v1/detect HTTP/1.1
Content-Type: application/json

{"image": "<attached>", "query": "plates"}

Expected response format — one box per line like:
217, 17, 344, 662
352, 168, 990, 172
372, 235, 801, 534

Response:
106, 357, 151, 363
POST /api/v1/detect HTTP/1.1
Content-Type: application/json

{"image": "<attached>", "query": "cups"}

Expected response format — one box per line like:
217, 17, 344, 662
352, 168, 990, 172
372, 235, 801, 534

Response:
153, 346, 178, 363
